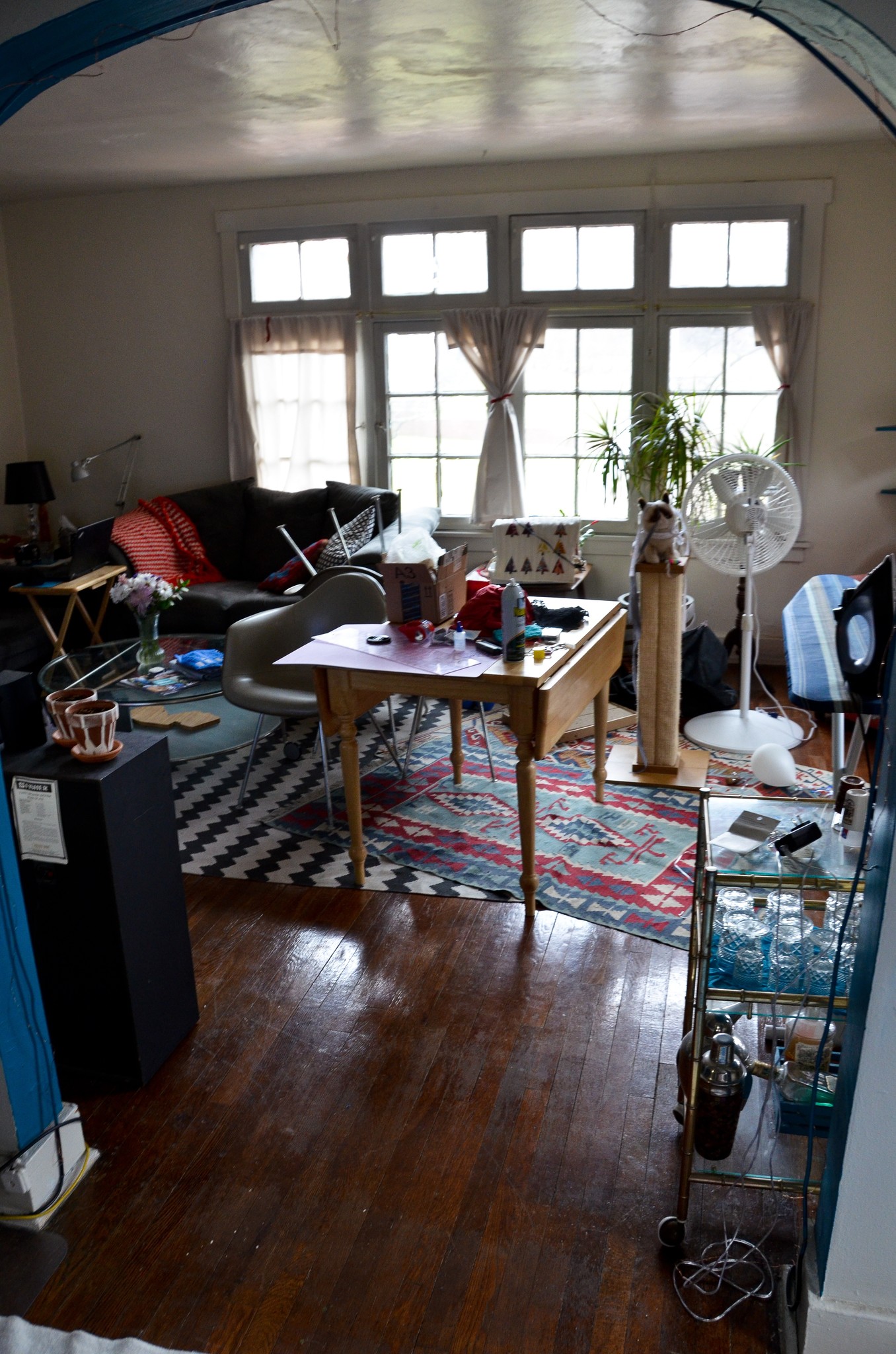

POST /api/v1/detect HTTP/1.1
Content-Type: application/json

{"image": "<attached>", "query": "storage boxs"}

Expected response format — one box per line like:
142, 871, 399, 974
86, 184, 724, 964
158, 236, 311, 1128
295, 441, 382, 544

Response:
381, 542, 468, 625
773, 1045, 842, 1138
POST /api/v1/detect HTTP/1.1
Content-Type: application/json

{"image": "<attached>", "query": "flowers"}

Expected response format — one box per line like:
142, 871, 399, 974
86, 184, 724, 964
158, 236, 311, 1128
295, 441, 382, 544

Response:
108, 571, 191, 666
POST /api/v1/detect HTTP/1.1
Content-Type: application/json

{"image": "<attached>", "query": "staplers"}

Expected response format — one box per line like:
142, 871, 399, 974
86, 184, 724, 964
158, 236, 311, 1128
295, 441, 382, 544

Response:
475, 640, 503, 655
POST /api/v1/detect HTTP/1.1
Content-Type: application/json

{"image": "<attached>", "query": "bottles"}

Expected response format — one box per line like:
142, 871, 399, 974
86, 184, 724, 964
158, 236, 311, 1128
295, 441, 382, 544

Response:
501, 578, 525, 663
453, 621, 466, 651
676, 1006, 749, 1098
749, 1058, 837, 1107
783, 1002, 835, 1074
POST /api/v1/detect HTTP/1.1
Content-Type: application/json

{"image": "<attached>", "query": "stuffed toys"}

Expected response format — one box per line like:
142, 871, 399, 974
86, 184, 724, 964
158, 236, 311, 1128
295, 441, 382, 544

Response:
637, 493, 680, 564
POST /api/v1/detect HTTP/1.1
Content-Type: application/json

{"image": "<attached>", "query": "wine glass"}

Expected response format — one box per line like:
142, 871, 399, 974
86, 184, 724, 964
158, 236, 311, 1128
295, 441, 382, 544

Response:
769, 925, 806, 995
803, 929, 839, 996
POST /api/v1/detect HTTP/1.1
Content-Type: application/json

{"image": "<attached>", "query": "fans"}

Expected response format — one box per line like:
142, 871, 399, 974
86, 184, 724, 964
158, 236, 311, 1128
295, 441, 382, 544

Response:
680, 455, 817, 753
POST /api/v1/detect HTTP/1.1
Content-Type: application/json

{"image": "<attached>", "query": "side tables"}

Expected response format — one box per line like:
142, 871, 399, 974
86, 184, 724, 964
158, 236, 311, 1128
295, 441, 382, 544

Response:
8, 565, 128, 699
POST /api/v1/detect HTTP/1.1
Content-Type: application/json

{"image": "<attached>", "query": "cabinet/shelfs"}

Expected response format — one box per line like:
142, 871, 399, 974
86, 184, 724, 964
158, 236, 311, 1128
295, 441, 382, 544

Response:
657, 788, 878, 1258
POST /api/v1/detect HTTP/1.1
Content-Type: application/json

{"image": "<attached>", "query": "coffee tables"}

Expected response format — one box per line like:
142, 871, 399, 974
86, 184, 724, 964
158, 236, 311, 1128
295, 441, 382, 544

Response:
37, 632, 284, 765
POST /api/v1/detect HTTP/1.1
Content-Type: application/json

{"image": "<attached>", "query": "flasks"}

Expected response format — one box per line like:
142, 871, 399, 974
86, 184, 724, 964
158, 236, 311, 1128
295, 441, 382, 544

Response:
695, 1033, 748, 1161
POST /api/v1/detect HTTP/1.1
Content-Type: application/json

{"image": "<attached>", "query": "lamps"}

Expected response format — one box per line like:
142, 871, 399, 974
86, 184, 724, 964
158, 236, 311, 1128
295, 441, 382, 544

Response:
3, 460, 58, 586
70, 435, 144, 518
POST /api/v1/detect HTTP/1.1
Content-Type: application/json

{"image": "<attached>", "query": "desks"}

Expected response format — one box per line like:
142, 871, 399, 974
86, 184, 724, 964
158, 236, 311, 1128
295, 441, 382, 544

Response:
781, 574, 871, 804
313, 596, 628, 917
465, 560, 594, 600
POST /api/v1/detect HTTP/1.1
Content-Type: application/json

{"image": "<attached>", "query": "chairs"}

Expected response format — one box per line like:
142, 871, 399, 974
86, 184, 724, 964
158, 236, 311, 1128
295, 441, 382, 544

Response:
221, 572, 405, 832
297, 566, 399, 764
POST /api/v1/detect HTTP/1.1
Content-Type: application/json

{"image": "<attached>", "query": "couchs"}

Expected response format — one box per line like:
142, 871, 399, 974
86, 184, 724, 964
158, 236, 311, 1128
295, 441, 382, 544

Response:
52, 484, 396, 651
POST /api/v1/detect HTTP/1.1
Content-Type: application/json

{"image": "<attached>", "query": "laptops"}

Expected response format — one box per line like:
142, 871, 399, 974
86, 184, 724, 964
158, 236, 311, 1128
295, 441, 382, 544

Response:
21, 516, 117, 582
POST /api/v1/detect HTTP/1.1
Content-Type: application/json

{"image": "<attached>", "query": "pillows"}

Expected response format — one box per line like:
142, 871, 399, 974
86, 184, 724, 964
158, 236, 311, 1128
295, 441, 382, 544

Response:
256, 538, 329, 592
313, 504, 376, 573
324, 481, 396, 541
163, 477, 255, 581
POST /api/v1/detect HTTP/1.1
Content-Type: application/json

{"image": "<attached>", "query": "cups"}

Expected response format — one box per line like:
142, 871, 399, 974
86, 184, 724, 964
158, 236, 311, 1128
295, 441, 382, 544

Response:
824, 898, 839, 931
831, 775, 871, 848
711, 887, 755, 936
732, 920, 770, 990
762, 891, 805, 941
828, 891, 864, 904
768, 913, 816, 980
715, 909, 758, 976
834, 906, 862, 998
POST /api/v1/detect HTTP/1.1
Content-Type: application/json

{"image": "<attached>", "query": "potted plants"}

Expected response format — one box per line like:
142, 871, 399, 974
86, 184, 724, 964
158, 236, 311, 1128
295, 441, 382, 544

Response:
567, 374, 810, 680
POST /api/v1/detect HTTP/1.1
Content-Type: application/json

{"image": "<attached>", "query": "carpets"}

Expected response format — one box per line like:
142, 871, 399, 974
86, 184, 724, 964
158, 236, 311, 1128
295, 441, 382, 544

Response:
258, 710, 879, 953
169, 689, 528, 902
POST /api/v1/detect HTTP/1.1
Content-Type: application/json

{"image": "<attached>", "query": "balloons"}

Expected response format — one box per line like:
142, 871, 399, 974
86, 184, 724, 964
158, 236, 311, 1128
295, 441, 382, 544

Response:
750, 743, 797, 787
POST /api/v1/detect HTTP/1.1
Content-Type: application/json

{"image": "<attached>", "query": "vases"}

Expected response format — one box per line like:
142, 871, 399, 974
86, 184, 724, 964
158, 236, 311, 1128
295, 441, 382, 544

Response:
135, 614, 165, 665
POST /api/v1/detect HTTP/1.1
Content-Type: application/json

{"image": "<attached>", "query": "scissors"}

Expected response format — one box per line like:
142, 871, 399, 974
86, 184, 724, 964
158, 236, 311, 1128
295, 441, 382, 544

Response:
544, 643, 560, 655
